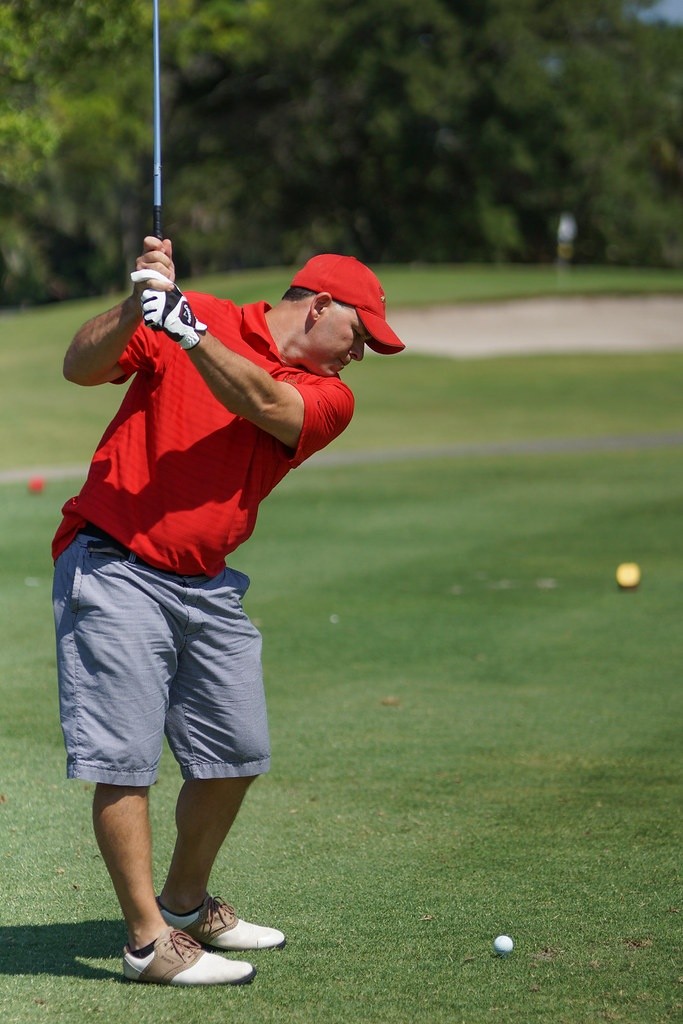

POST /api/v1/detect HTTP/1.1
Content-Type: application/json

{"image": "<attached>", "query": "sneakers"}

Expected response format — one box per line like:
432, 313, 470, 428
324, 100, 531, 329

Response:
123, 926, 257, 986
155, 893, 286, 951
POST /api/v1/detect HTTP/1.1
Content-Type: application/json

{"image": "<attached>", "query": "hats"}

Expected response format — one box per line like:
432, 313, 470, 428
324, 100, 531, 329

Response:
289, 253, 406, 355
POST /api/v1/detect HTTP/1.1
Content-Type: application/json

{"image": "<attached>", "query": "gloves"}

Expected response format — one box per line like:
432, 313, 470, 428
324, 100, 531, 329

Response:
130, 269, 208, 350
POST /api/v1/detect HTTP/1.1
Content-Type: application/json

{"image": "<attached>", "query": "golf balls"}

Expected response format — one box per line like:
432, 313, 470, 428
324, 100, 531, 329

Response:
494, 935, 514, 955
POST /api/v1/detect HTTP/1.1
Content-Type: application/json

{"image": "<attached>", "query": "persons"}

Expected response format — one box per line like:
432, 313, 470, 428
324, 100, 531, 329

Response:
51, 236, 406, 986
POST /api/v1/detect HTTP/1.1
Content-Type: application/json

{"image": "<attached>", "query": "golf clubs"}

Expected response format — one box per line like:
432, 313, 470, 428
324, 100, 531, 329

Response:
153, 1, 162, 242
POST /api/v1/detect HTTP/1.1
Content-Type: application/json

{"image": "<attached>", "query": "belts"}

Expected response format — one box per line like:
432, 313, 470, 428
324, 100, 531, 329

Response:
78, 522, 206, 577
